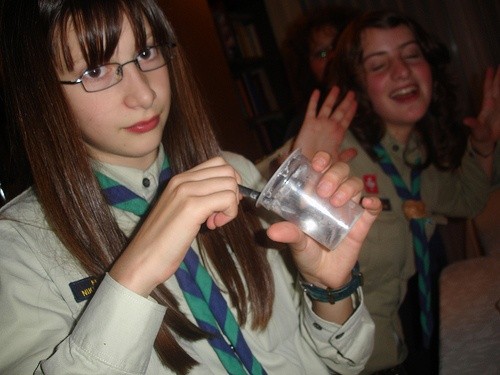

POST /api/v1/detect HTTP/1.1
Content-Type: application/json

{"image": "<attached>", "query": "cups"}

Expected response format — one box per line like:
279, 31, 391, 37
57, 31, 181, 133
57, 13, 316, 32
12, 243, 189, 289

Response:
256, 148, 365, 251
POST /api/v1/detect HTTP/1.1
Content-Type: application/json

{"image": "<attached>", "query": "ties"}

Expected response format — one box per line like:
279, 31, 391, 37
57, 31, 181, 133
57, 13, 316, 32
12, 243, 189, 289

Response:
372, 142, 434, 347
93, 155, 266, 375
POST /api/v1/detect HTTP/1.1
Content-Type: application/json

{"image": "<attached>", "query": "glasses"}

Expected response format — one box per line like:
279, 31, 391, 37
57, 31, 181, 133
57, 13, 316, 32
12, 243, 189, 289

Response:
59, 44, 172, 93
310, 48, 329, 59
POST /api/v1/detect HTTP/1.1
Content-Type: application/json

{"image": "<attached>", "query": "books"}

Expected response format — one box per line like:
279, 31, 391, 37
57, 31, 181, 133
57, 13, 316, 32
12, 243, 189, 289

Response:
217, 11, 288, 154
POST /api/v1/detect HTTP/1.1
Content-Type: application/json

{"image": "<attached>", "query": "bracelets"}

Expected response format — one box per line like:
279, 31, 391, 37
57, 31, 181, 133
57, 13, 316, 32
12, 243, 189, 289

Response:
297, 268, 364, 305
471, 141, 496, 158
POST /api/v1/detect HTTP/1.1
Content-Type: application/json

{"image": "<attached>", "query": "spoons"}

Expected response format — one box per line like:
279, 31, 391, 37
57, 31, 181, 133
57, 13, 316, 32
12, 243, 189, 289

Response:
238, 184, 340, 244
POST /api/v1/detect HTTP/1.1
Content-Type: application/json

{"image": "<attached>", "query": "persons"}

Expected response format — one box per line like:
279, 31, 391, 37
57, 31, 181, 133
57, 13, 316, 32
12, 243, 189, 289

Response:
0, 0, 382, 375
288, 17, 449, 375
253, 4, 500, 375
